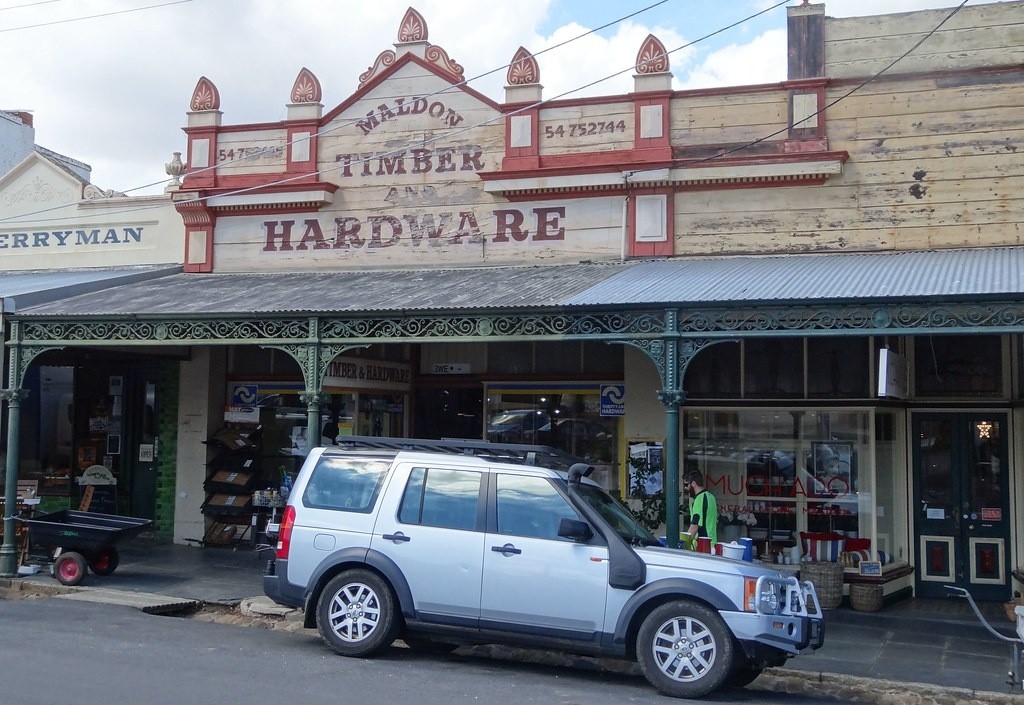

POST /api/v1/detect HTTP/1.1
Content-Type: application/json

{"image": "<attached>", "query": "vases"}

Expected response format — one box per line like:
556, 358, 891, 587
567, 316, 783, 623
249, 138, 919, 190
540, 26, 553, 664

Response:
723, 525, 741, 542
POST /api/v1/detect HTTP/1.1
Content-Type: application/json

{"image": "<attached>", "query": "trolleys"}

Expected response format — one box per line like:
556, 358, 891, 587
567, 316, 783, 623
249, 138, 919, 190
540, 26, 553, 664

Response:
11, 508, 155, 587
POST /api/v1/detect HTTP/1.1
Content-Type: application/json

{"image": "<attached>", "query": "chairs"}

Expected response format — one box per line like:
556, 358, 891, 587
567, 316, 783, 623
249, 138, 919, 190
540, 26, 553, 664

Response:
0, 480, 39, 566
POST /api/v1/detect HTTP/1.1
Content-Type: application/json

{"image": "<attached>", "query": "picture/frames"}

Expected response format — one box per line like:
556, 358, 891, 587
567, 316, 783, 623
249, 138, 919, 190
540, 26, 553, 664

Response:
810, 441, 854, 498
623, 438, 666, 500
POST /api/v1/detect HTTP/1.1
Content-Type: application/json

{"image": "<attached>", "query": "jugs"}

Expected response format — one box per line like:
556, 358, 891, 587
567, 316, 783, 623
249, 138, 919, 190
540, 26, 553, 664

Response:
791, 546, 800, 564
738, 537, 753, 562
716, 542, 726, 555
659, 536, 667, 547
697, 536, 712, 553
680, 532, 691, 549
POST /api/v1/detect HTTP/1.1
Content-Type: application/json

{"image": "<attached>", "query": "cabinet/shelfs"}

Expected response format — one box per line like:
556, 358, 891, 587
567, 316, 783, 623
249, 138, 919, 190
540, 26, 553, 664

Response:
199, 381, 409, 548
482, 381, 622, 491
747, 458, 859, 548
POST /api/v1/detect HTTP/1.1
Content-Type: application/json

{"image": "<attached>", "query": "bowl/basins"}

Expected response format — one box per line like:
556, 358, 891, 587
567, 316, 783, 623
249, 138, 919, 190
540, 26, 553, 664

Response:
756, 479, 764, 483
773, 477, 783, 484
722, 541, 746, 560
749, 478, 754, 483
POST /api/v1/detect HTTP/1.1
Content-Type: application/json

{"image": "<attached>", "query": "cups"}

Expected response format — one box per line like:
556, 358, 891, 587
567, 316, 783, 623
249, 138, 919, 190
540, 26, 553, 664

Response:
778, 556, 783, 563
785, 557, 790, 564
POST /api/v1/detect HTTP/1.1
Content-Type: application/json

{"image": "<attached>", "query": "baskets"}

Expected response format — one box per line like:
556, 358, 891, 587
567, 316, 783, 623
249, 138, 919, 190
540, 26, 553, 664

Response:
850, 582, 883, 611
800, 556, 845, 611
1005, 593, 1024, 621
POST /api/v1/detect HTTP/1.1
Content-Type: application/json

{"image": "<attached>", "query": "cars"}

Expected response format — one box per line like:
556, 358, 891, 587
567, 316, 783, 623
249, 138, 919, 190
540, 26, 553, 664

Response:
488, 404, 616, 469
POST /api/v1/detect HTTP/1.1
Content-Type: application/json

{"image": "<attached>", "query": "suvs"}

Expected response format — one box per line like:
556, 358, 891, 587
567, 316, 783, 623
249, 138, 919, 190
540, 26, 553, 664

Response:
261, 437, 827, 699
681, 436, 835, 525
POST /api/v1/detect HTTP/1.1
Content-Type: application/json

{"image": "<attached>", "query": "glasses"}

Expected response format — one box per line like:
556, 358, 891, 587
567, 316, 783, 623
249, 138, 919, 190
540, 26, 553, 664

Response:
684, 480, 692, 487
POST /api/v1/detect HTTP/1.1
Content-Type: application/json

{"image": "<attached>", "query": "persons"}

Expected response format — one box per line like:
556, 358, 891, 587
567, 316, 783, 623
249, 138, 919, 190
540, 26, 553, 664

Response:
682, 468, 718, 551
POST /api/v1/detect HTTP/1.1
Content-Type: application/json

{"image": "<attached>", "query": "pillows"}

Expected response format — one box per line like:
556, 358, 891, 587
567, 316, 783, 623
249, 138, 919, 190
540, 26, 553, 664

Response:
798, 532, 893, 566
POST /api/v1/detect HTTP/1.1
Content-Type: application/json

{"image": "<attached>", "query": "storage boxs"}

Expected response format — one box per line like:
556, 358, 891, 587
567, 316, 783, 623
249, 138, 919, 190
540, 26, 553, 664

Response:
1003, 593, 1024, 621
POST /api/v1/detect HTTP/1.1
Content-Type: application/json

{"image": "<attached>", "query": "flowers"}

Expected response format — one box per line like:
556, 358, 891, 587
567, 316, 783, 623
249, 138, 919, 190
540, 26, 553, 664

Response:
717, 509, 758, 530
